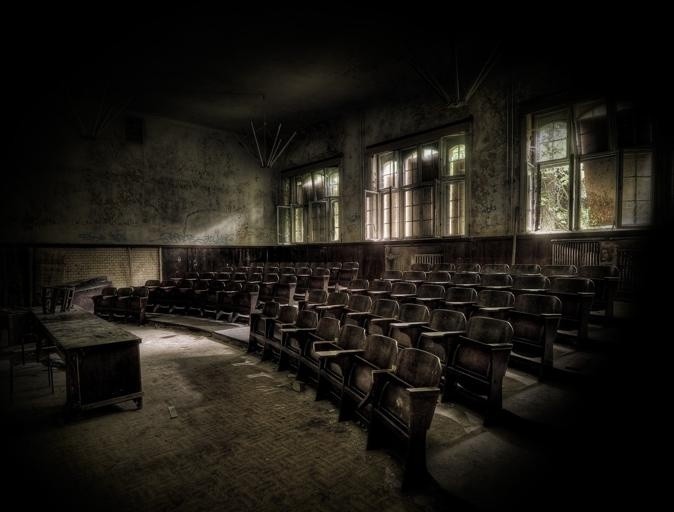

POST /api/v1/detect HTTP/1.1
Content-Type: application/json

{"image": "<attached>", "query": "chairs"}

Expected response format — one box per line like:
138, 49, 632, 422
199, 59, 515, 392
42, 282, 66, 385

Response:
9, 330, 58, 404
91, 262, 625, 482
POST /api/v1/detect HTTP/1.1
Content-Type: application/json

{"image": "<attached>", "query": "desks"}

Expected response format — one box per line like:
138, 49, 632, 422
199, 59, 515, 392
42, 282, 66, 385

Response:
30, 302, 146, 425
